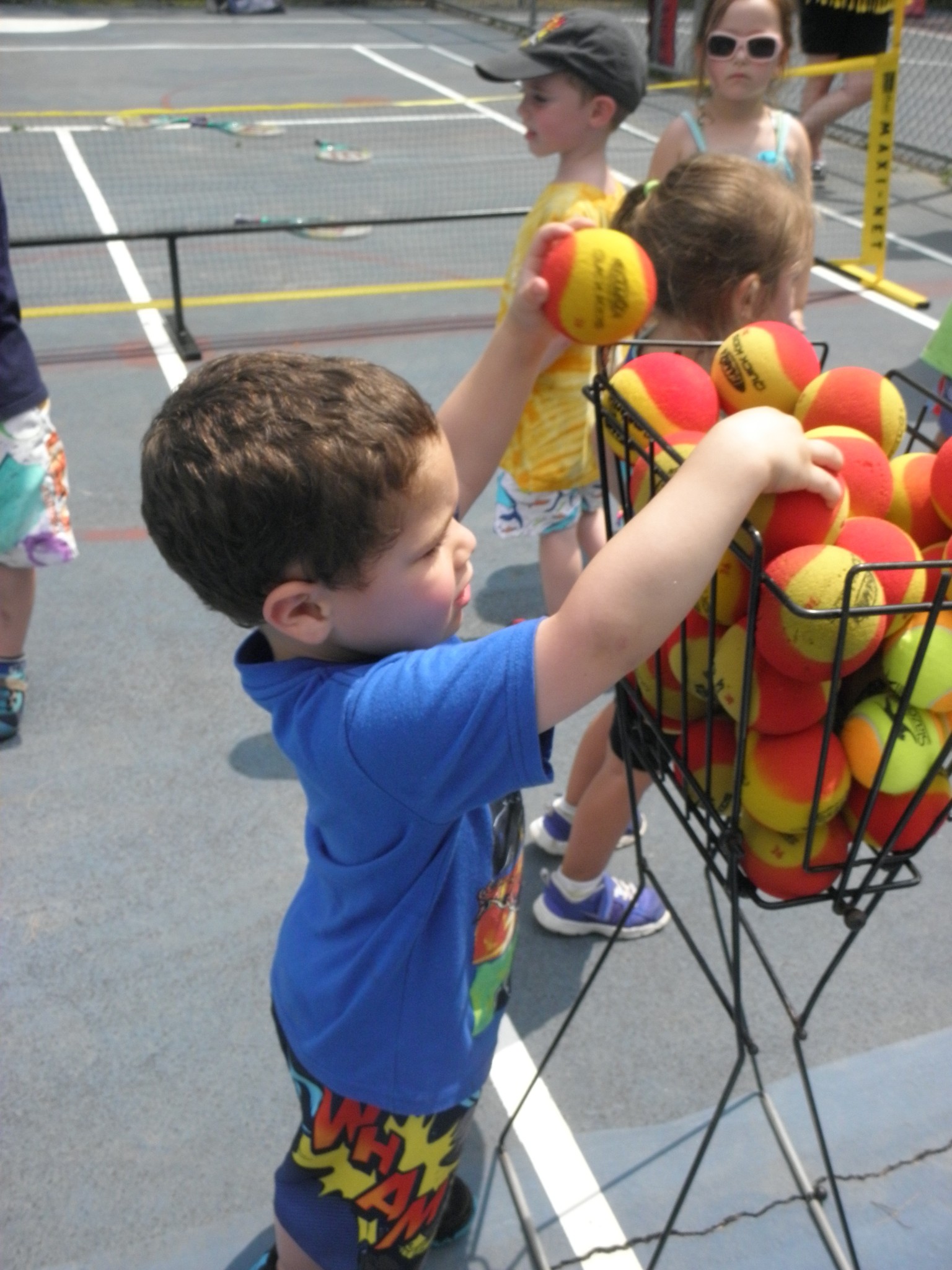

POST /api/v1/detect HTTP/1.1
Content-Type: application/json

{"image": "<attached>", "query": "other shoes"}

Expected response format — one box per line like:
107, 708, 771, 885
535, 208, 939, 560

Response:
812, 158, 831, 187
430, 1178, 479, 1247
0, 671, 30, 739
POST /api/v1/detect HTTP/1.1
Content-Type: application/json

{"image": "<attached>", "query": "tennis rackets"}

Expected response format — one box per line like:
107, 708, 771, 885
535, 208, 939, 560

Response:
104, 114, 205, 129
234, 212, 374, 240
315, 137, 377, 164
191, 118, 288, 139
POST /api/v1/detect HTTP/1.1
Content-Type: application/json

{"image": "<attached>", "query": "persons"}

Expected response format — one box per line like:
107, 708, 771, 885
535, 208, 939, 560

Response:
142, 217, 843, 1269
529, 152, 810, 935
0, 185, 78, 742
473, 11, 648, 621
796, 1, 924, 187
649, 1, 814, 210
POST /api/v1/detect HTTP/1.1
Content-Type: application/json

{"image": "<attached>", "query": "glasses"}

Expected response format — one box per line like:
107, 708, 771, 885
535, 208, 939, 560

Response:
704, 30, 786, 62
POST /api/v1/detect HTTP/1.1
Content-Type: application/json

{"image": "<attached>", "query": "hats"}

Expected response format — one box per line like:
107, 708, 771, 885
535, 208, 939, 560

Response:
474, 8, 649, 113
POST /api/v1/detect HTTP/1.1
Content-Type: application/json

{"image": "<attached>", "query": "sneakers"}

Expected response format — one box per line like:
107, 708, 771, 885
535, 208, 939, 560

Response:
531, 795, 649, 857
533, 872, 670, 940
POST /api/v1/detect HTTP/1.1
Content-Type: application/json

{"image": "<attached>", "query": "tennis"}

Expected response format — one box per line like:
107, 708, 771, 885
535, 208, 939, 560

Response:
796, 366, 909, 457
600, 350, 721, 463
624, 425, 952, 898
711, 320, 820, 417
541, 226, 657, 344
